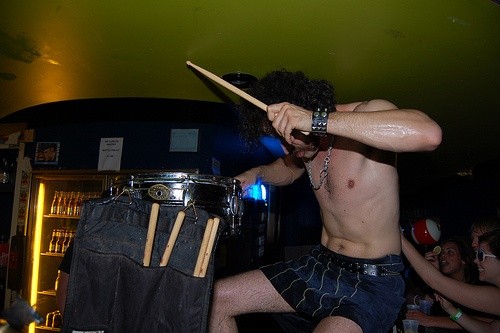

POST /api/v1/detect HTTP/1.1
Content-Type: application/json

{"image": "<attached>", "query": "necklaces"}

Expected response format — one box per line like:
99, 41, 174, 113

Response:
308, 138, 335, 190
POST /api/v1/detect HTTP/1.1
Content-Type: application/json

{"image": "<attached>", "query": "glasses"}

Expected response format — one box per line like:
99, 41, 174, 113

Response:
474, 250, 498, 263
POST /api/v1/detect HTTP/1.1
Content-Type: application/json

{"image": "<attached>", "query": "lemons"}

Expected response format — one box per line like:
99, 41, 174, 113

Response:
433, 246, 441, 255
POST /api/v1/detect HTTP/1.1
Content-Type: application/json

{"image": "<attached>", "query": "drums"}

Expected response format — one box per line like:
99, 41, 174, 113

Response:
111, 172, 244, 234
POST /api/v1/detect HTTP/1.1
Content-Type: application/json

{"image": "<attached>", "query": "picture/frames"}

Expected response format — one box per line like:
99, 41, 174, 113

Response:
34, 142, 60, 165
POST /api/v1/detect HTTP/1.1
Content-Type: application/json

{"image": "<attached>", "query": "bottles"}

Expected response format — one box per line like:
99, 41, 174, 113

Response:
51, 190, 101, 215
54, 269, 60, 291
48, 229, 76, 254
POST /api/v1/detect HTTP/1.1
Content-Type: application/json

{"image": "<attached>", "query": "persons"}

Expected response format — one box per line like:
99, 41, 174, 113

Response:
206, 69, 443, 333
425, 238, 479, 286
399, 229, 500, 333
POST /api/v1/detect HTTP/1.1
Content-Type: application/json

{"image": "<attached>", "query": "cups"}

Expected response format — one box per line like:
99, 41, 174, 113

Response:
419, 300, 432, 314
407, 305, 420, 310
402, 320, 418, 333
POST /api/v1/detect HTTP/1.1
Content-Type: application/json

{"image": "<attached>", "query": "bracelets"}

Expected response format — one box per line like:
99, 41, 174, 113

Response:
311, 107, 328, 136
449, 308, 462, 321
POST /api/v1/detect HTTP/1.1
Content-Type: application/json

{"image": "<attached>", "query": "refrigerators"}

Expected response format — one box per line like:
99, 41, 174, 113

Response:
23, 168, 120, 333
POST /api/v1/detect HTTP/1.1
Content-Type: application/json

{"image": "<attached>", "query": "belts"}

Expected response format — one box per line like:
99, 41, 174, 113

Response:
315, 243, 406, 276
